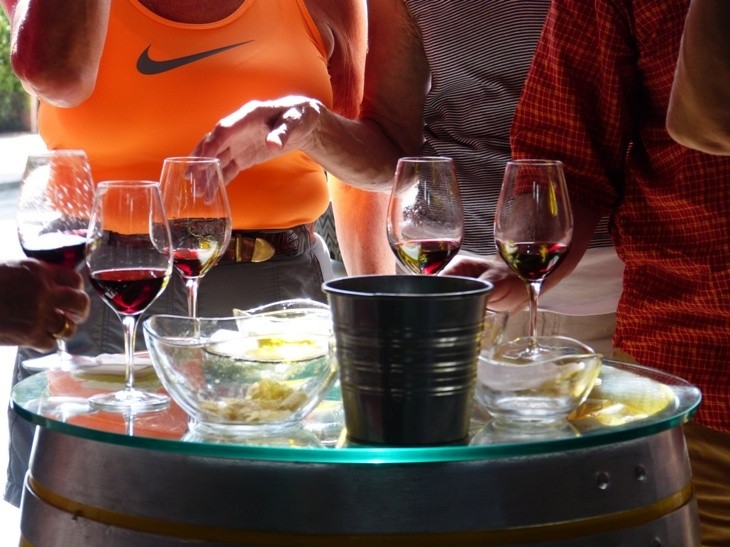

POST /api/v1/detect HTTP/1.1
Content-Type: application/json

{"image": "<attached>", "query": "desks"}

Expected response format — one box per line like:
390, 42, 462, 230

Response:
10, 360, 713, 547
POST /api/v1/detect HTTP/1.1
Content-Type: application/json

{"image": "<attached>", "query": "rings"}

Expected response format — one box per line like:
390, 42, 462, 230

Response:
52, 321, 69, 338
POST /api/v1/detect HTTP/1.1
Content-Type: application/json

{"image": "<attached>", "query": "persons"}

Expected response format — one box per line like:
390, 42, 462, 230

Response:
182, 0, 625, 360
0, 258, 89, 353
437, 0, 730, 547
0, 0, 396, 512
666, 1, 730, 156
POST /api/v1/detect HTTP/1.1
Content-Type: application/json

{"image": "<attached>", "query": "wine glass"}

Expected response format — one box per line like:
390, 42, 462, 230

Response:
494, 160, 573, 356
16, 150, 96, 369
85, 179, 173, 413
149, 157, 231, 336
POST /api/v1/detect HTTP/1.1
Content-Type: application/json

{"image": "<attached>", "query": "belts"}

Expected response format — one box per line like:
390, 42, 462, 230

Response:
102, 221, 321, 264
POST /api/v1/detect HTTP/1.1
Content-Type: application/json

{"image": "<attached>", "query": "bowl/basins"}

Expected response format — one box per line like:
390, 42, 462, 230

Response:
234, 299, 333, 335
141, 313, 338, 436
474, 335, 600, 419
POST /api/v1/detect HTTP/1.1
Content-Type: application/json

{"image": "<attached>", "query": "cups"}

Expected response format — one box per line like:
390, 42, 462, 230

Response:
387, 155, 463, 275
483, 312, 508, 346
321, 275, 494, 446
